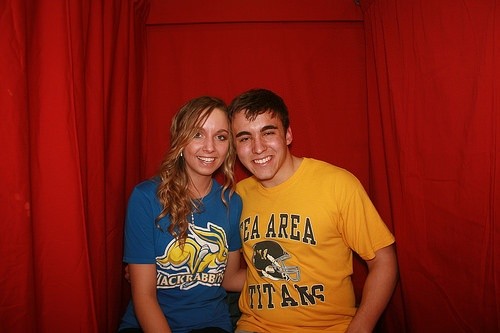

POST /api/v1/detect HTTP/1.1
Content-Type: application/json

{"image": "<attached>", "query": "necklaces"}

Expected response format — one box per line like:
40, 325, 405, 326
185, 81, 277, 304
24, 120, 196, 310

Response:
181, 179, 213, 233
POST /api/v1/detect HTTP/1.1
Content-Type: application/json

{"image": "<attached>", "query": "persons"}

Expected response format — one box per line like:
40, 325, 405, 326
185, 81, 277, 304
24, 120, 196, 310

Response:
116, 95, 249, 333
122, 88, 401, 333
